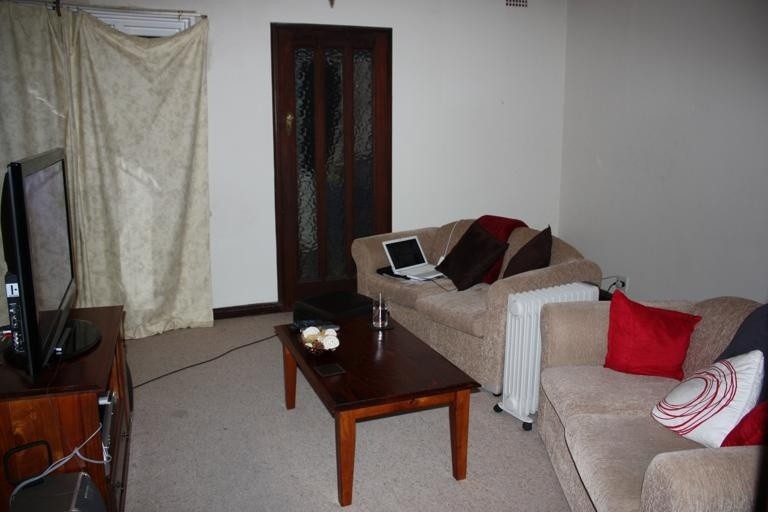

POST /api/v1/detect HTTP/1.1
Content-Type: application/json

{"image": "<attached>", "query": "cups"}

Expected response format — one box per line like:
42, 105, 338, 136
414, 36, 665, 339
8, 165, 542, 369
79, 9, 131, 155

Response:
372, 291, 391, 326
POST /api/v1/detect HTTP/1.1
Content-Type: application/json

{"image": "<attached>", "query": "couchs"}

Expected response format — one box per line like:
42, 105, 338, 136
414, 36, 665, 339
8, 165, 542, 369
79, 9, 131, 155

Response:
347, 213, 604, 395
534, 294, 768, 512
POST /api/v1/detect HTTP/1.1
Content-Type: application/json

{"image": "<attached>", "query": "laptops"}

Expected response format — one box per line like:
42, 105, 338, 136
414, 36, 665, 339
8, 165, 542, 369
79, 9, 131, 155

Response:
382, 236, 442, 280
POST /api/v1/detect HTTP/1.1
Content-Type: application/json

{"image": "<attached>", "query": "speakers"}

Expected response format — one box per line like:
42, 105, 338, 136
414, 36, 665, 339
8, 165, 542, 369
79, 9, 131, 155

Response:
9, 472, 107, 512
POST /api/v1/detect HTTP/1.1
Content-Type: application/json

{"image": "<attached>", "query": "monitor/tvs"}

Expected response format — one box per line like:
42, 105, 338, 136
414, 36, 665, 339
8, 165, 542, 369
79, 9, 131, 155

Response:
0, 147, 102, 377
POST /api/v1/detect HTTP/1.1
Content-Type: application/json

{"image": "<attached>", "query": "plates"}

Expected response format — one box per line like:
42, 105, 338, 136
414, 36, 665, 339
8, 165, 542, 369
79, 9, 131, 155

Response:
298, 332, 343, 356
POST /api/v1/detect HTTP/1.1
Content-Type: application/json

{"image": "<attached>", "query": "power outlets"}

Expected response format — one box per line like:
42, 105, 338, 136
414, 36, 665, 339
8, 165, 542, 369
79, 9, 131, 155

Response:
614, 273, 630, 292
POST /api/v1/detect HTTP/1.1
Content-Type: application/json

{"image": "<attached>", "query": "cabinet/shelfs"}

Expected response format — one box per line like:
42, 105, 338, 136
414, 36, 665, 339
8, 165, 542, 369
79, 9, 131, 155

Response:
0, 303, 132, 510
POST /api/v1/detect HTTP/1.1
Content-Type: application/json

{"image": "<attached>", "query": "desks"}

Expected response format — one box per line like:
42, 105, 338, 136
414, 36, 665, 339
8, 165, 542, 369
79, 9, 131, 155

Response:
274, 308, 482, 507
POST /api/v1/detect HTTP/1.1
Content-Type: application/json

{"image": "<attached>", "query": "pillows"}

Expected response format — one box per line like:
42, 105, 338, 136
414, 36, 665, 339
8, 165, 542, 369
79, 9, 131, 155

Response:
504, 224, 554, 278
650, 347, 764, 446
603, 287, 701, 384
720, 398, 767, 447
435, 219, 510, 290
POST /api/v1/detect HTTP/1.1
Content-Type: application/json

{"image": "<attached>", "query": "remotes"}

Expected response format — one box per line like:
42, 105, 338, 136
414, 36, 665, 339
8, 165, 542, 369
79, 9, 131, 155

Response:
299, 325, 340, 333
294, 319, 324, 328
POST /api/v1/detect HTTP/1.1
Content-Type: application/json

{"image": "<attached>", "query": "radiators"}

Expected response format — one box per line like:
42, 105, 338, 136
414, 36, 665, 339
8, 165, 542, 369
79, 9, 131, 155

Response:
491, 280, 600, 430
